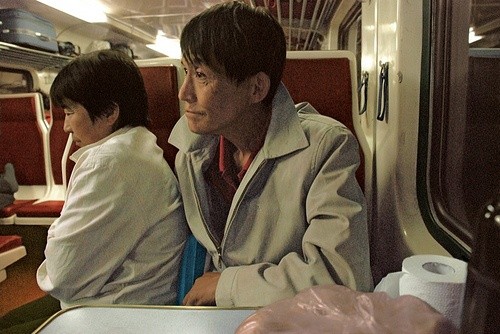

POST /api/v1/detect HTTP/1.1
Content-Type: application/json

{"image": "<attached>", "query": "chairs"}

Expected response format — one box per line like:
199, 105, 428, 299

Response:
14, 95, 69, 225
0, 235, 28, 283
61, 57, 186, 194
0, 92, 50, 225
282, 48, 373, 230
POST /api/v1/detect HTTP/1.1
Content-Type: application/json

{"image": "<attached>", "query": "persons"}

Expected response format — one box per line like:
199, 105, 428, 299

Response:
0, 163, 18, 208
0, 48, 190, 334
168, 0, 370, 309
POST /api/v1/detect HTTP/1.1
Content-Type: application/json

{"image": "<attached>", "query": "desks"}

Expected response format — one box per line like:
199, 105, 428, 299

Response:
32, 304, 263, 334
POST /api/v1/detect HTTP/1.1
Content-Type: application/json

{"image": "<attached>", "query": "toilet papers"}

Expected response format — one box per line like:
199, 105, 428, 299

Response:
372, 254, 469, 330
